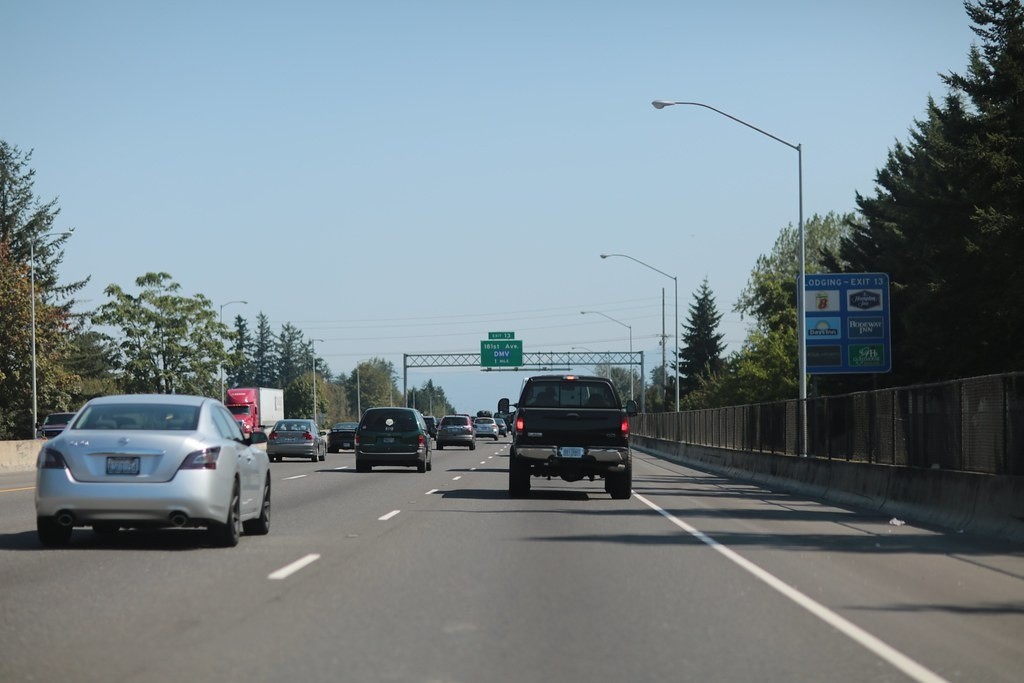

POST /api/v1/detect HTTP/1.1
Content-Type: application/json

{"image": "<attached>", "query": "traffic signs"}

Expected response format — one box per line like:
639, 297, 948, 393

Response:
480, 332, 523, 367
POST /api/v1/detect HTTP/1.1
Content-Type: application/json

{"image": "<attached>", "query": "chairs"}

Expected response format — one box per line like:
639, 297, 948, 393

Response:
535, 392, 558, 407
582, 393, 609, 408
166, 419, 187, 431
94, 419, 118, 429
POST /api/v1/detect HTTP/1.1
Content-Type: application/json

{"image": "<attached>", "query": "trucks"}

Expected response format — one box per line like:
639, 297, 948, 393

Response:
225, 386, 285, 444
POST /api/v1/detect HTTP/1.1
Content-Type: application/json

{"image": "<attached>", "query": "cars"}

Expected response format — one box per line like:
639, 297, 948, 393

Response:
325, 422, 360, 454
266, 419, 326, 462
35, 412, 77, 441
34, 394, 273, 547
493, 417, 512, 437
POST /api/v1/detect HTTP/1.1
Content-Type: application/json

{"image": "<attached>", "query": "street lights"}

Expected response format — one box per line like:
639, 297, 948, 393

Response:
30, 231, 74, 439
600, 253, 680, 416
580, 310, 634, 402
312, 339, 325, 424
219, 300, 248, 403
356, 359, 372, 423
650, 100, 809, 462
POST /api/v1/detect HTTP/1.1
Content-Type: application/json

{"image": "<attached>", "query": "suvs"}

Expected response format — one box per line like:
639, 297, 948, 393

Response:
473, 416, 501, 441
354, 406, 434, 473
435, 415, 477, 450
423, 415, 443, 441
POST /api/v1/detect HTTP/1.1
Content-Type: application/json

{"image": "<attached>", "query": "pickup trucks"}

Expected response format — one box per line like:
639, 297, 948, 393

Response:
497, 373, 640, 500
